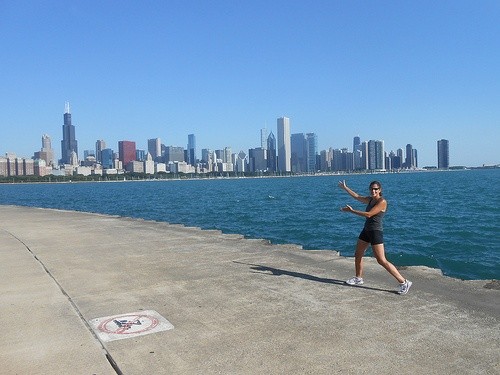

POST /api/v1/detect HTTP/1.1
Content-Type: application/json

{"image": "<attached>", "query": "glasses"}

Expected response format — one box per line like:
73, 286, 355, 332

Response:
371, 188, 380, 191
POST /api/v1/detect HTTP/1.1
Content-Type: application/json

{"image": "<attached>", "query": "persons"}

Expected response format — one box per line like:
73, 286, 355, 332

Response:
338, 179, 412, 295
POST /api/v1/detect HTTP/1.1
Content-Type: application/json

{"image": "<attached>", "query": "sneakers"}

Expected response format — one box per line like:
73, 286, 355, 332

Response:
345, 276, 363, 285
397, 279, 413, 295
